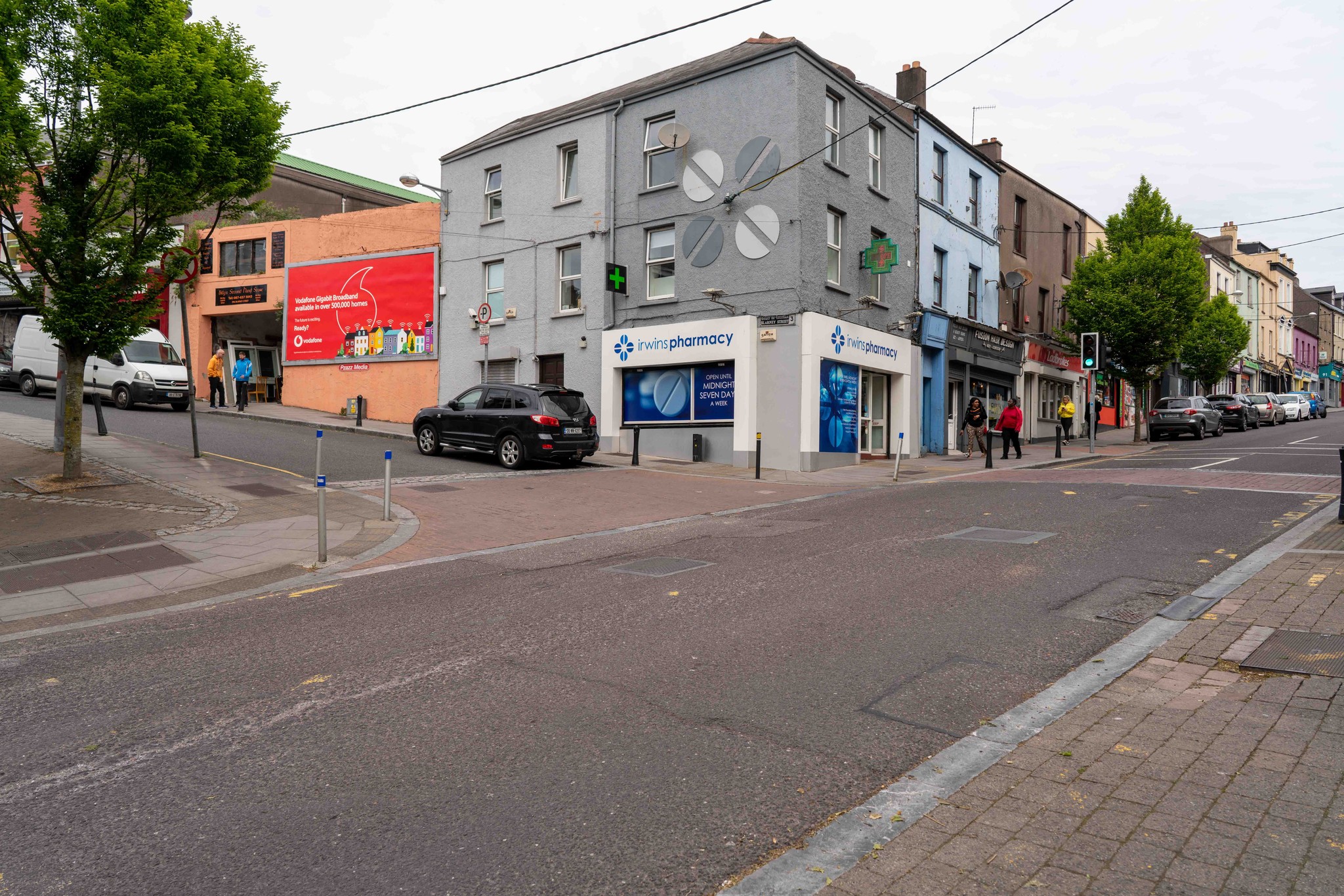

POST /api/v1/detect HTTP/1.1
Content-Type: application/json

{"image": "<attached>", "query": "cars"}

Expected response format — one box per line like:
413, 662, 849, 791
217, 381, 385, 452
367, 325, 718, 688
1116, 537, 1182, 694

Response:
0, 344, 44, 392
1275, 393, 1311, 422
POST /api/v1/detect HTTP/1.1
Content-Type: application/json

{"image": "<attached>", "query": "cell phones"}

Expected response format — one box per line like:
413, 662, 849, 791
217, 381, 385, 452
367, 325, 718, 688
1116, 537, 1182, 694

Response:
1062, 407, 1065, 409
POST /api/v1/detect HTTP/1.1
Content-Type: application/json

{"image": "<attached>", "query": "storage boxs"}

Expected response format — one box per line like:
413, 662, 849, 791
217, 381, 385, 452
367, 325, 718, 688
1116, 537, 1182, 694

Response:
562, 298, 580, 310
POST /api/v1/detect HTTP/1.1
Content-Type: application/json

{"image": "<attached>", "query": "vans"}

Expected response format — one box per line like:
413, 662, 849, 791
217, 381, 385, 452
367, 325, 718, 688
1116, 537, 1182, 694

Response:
8, 315, 197, 412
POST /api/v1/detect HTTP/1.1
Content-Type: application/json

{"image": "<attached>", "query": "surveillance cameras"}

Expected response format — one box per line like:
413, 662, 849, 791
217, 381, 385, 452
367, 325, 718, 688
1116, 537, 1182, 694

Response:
468, 308, 477, 318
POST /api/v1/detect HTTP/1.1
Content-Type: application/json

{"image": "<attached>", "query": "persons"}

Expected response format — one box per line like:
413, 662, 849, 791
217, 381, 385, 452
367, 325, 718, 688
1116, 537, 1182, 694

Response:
207, 349, 229, 409
1084, 395, 1102, 440
1245, 387, 1251, 393
231, 352, 252, 408
959, 397, 986, 458
1058, 395, 1075, 446
1300, 388, 1304, 391
994, 398, 1023, 459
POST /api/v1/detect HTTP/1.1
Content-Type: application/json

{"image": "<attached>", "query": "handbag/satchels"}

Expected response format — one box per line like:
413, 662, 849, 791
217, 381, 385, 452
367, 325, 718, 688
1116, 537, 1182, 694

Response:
983, 426, 987, 436
1080, 420, 1088, 436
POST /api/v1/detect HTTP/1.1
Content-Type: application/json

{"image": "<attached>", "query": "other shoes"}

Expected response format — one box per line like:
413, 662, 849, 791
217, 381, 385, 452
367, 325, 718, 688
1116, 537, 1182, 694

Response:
1088, 437, 1090, 439
1095, 437, 1096, 440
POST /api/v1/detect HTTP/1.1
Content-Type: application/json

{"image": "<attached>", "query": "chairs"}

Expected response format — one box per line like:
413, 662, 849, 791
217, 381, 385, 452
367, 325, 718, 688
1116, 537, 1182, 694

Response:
486, 395, 504, 409
516, 393, 531, 409
248, 376, 268, 403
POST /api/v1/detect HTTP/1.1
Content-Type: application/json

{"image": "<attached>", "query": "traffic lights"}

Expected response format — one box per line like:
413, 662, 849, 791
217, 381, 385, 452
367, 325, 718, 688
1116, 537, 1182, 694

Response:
1099, 334, 1111, 369
1081, 332, 1099, 370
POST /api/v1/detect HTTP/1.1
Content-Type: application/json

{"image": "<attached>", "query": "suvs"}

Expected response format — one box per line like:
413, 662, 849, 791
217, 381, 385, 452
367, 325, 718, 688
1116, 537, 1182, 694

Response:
1245, 392, 1287, 426
413, 381, 599, 470
1205, 393, 1261, 432
1288, 390, 1328, 419
1148, 396, 1224, 442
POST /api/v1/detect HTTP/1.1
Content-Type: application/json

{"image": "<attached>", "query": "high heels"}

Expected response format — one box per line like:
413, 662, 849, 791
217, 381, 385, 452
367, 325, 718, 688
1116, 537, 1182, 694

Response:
967, 454, 972, 458
980, 452, 987, 458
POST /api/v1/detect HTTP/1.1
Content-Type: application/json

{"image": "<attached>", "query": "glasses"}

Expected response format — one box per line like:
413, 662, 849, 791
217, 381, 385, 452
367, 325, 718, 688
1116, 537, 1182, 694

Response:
220, 352, 225, 356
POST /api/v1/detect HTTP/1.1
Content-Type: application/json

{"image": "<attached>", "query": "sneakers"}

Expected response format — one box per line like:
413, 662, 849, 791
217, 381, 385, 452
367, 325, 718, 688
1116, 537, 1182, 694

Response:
218, 404, 229, 408
1016, 454, 1022, 459
232, 404, 240, 408
1000, 455, 1008, 459
1062, 440, 1067, 445
209, 405, 217, 409
1066, 440, 1070, 445
245, 404, 248, 408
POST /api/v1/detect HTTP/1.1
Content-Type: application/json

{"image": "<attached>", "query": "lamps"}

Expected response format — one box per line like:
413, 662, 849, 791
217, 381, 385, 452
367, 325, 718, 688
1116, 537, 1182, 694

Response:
399, 172, 452, 215
1057, 367, 1067, 371
1211, 290, 1243, 299
1289, 312, 1317, 327
1280, 353, 1295, 362
